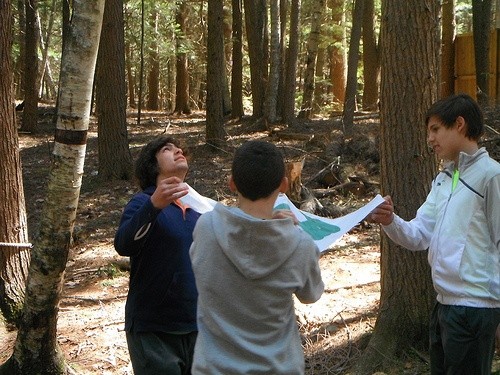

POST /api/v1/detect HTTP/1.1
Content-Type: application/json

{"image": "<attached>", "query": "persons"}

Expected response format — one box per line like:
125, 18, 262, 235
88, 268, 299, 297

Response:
111, 139, 205, 375
188, 140, 325, 375
370, 93, 500, 375
